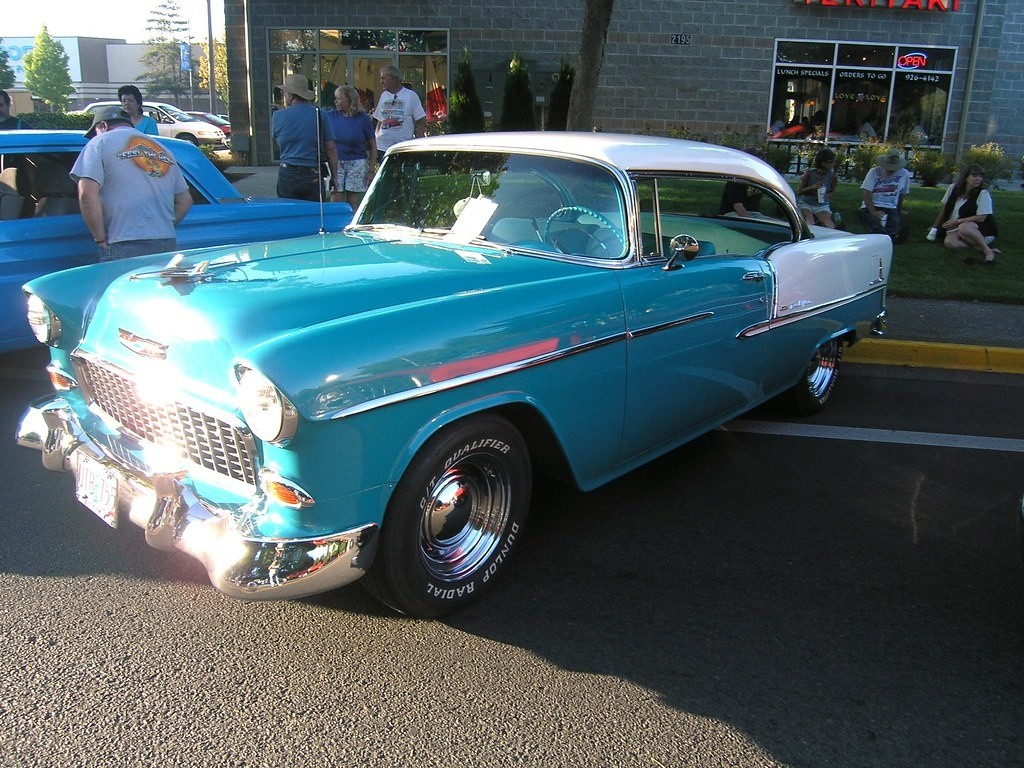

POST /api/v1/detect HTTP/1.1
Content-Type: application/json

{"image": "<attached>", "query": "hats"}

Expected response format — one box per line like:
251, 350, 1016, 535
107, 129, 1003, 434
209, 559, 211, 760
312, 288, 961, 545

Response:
276, 74, 315, 101
878, 149, 906, 170
84, 107, 132, 141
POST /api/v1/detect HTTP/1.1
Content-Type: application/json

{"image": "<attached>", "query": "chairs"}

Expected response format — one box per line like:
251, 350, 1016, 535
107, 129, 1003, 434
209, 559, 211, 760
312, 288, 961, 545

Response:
586, 225, 715, 260
493, 217, 599, 260
0, 164, 81, 220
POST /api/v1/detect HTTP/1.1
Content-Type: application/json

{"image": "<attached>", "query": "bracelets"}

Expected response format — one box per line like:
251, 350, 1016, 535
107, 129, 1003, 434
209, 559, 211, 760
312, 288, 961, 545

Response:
958, 219, 960, 223
95, 240, 106, 243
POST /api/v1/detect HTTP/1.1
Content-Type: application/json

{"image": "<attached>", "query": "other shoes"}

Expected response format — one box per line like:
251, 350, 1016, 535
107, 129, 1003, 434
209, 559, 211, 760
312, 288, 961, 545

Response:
983, 253, 996, 266
893, 225, 910, 244
835, 223, 846, 232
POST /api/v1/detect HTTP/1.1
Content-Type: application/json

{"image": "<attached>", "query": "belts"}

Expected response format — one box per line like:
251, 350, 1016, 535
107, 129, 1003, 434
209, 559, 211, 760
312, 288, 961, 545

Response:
280, 163, 314, 171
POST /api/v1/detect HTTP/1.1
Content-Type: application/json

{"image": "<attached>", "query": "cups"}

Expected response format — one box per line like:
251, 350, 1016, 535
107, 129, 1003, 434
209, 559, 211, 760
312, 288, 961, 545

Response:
835, 213, 841, 220
880, 214, 888, 227
927, 228, 937, 241
818, 187, 826, 203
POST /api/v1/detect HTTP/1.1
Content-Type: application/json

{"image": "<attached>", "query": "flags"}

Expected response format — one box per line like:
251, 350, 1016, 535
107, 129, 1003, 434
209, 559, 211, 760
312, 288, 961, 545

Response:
426, 48, 447, 123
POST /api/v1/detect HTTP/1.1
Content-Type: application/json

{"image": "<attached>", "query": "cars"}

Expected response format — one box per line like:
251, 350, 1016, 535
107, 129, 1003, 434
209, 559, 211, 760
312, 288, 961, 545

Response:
0, 126, 354, 354
81, 101, 231, 148
14, 128, 895, 622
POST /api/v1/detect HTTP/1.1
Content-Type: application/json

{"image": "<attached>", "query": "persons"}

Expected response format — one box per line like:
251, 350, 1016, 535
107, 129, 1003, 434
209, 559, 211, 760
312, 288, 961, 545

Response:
0, 90, 32, 129
373, 67, 426, 168
800, 148, 846, 230
771, 111, 928, 145
271, 73, 339, 202
326, 85, 377, 214
118, 85, 159, 136
719, 147, 783, 221
860, 149, 910, 243
69, 107, 193, 264
927, 164, 998, 264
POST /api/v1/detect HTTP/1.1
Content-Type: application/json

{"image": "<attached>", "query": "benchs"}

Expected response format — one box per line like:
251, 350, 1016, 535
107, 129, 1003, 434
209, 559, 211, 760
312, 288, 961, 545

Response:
577, 213, 792, 257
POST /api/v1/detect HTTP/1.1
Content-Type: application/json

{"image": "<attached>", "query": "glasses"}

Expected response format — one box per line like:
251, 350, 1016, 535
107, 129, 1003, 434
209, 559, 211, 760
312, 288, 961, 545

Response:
392, 94, 397, 105
